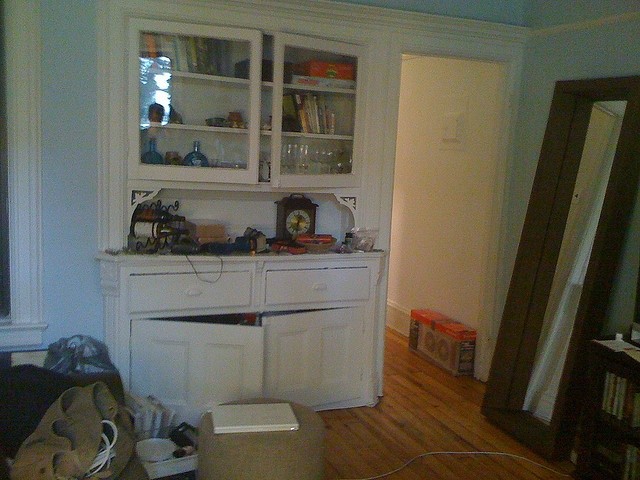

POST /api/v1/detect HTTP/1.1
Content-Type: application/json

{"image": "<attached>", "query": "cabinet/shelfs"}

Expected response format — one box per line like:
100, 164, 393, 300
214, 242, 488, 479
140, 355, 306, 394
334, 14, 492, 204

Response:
120, 9, 373, 426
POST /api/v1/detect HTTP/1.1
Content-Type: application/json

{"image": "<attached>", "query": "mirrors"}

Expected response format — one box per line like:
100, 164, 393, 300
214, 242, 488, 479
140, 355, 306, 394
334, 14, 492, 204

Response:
481, 75, 639, 464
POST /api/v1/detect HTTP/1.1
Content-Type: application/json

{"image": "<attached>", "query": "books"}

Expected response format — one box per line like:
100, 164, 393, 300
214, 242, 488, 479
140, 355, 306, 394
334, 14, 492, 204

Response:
601, 369, 640, 429
283, 92, 336, 135
591, 438, 640, 480
142, 35, 233, 77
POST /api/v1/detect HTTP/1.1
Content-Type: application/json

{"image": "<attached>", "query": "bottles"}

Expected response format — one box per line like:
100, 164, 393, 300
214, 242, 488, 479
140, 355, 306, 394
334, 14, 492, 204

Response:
134, 210, 185, 224
133, 221, 189, 240
141, 138, 163, 165
183, 141, 208, 166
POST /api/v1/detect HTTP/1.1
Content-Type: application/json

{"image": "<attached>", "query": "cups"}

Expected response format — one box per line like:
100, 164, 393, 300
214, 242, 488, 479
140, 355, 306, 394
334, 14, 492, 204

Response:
166, 152, 179, 165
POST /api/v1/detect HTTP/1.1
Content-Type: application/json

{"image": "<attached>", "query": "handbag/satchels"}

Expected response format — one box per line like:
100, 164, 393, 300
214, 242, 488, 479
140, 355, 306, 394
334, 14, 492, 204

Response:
11, 383, 136, 480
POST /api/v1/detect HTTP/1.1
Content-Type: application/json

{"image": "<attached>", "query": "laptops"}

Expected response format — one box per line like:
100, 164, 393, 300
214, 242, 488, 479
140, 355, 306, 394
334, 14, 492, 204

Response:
209, 402, 299, 435
